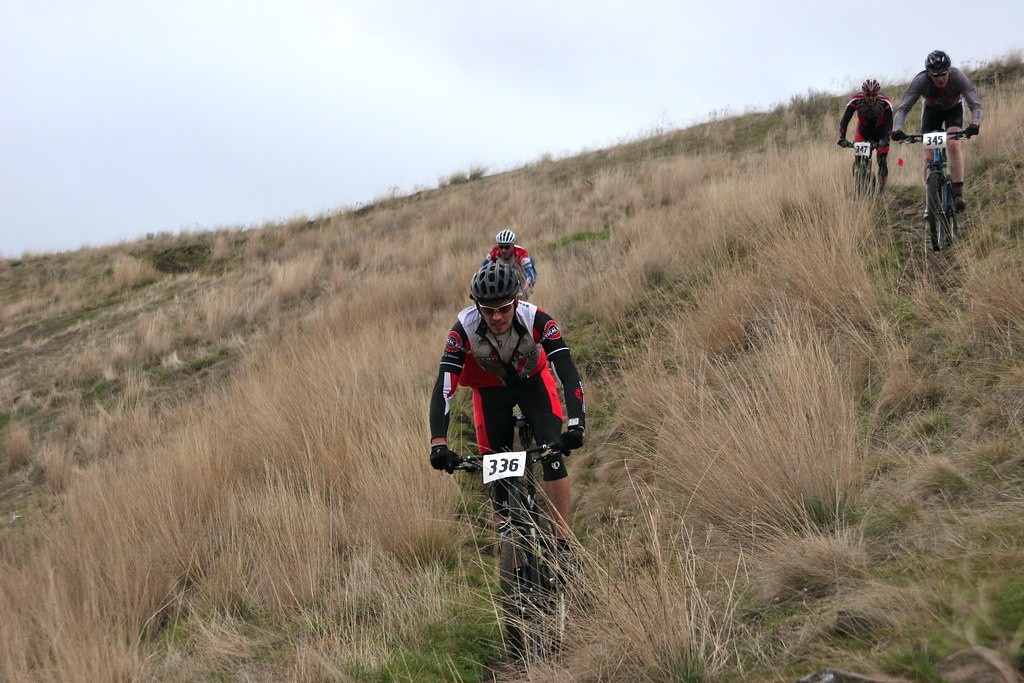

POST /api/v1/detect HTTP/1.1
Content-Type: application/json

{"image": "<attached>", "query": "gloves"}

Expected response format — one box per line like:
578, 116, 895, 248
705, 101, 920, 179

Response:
892, 130, 905, 142
559, 427, 583, 456
430, 442, 458, 475
965, 124, 979, 139
879, 138, 889, 147
838, 138, 849, 148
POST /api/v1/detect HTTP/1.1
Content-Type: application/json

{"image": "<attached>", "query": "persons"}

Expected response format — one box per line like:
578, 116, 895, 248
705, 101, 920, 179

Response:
892, 49, 982, 212
837, 81, 894, 196
429, 260, 586, 584
482, 230, 537, 302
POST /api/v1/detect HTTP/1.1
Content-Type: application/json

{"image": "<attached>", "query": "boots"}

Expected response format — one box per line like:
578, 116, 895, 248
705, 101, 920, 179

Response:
951, 181, 966, 214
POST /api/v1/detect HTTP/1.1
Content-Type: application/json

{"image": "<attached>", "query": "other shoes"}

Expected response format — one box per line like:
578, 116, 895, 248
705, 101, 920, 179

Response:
557, 537, 584, 583
513, 563, 529, 620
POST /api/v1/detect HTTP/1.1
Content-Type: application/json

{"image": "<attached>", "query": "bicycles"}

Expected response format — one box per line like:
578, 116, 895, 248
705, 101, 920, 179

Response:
837, 141, 889, 196
902, 128, 979, 252
448, 412, 584, 677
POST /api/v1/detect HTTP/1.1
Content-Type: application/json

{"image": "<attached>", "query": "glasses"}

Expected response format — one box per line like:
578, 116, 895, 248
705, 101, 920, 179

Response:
930, 68, 948, 77
499, 243, 511, 250
477, 299, 515, 316
864, 93, 878, 98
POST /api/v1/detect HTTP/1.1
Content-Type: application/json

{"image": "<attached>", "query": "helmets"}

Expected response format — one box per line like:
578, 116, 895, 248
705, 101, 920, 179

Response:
496, 229, 517, 244
925, 50, 950, 78
470, 262, 520, 303
862, 79, 881, 94
